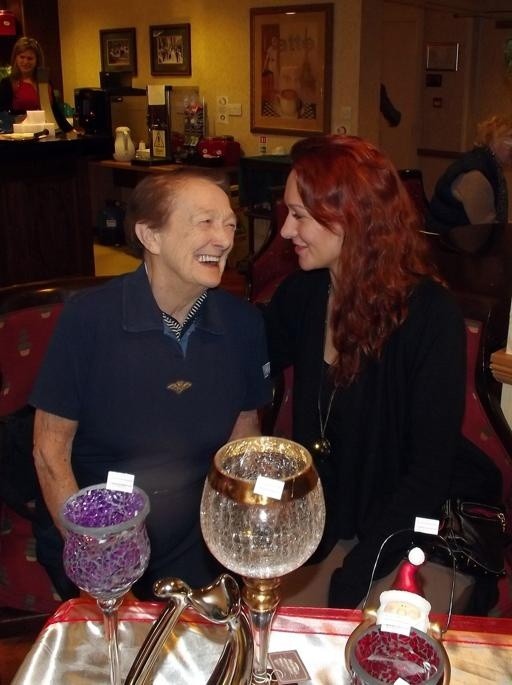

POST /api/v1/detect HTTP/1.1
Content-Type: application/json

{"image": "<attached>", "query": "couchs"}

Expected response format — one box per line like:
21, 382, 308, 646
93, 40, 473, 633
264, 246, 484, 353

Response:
1, 170, 511, 637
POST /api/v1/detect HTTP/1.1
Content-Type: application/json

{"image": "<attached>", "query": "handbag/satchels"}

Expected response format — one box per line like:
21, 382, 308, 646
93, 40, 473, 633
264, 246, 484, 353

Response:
436, 496, 507, 580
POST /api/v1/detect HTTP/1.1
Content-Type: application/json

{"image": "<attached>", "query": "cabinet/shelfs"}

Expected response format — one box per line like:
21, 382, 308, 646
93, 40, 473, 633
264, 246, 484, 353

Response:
0, 0, 64, 104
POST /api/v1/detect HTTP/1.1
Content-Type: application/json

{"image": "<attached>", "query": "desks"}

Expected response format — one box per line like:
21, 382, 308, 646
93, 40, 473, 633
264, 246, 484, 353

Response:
10, 598, 512, 685
89, 155, 299, 259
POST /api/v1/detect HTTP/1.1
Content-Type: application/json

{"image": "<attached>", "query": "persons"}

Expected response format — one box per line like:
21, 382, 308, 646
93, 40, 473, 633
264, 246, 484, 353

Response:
262, 133, 504, 617
430, 116, 512, 228
264, 35, 279, 90
30, 166, 279, 607
110, 45, 128, 62
159, 45, 182, 63
0, 36, 73, 141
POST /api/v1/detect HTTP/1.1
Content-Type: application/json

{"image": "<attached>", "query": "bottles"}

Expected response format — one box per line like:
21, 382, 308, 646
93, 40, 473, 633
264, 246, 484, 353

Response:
148, 119, 172, 161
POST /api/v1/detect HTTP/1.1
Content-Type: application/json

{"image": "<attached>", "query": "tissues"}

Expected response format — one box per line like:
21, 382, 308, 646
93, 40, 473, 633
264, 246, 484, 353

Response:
14, 110, 56, 137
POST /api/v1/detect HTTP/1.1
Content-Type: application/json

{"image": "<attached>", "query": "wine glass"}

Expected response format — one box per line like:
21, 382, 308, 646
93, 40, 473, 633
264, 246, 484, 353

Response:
57, 482, 150, 685
199, 437, 325, 685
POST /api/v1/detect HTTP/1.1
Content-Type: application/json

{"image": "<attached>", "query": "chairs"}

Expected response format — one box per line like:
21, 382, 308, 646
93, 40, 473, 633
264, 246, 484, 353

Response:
427, 141, 508, 259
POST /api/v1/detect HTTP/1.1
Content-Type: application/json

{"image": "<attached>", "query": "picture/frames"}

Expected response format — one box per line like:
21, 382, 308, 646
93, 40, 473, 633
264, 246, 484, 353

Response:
149, 22, 191, 76
99, 27, 137, 72
425, 41, 459, 72
250, 4, 333, 137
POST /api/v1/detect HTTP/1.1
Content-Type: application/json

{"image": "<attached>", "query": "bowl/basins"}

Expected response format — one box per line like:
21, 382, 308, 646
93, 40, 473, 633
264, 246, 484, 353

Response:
343, 628, 452, 685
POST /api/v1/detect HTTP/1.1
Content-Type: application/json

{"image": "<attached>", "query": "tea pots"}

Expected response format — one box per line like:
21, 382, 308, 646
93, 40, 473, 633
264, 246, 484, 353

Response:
112, 127, 135, 160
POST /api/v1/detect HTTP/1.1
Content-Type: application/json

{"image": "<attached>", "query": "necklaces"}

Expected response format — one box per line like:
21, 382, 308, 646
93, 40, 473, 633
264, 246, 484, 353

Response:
310, 360, 351, 458
